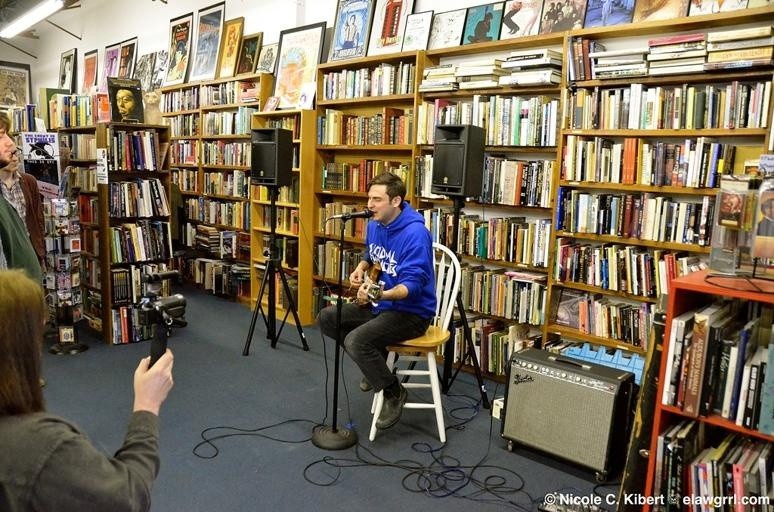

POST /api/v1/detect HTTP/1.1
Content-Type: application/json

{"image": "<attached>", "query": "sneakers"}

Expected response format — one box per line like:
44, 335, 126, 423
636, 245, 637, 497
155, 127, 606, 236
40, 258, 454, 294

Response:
358, 352, 400, 393
374, 376, 408, 430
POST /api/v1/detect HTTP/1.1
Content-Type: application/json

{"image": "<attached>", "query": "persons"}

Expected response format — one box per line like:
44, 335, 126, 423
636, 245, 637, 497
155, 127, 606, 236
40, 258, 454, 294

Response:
467, 13, 492, 43
544, 0, 582, 32
341, 11, 359, 49
504, 2, 521, 34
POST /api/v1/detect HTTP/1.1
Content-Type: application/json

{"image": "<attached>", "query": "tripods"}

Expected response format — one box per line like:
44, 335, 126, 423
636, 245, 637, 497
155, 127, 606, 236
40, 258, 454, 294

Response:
243, 187, 308, 356
400, 197, 492, 410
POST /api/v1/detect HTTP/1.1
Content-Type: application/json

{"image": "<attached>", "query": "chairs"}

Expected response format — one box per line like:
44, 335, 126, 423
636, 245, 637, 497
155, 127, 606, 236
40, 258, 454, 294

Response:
363, 240, 463, 442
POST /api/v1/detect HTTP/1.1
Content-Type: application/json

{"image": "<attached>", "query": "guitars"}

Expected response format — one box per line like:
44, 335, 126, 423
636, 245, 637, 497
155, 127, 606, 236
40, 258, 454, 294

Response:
347, 262, 383, 306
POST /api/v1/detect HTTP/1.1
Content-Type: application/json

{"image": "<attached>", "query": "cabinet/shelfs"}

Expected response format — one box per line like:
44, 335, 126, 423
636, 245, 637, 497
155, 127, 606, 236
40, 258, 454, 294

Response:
0, 3, 774, 387
642, 261, 774, 510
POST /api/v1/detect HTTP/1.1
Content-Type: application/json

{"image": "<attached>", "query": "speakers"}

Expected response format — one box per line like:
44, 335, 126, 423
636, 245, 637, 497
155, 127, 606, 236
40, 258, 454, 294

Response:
251, 128, 293, 187
431, 124, 486, 197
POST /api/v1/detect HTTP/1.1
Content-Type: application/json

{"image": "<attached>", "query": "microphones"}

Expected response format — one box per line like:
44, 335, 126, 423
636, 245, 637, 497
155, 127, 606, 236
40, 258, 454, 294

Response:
331, 209, 373, 219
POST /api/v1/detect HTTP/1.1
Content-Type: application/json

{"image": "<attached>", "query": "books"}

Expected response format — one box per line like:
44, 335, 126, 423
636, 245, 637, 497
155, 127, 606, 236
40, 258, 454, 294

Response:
0, 25, 774, 512
